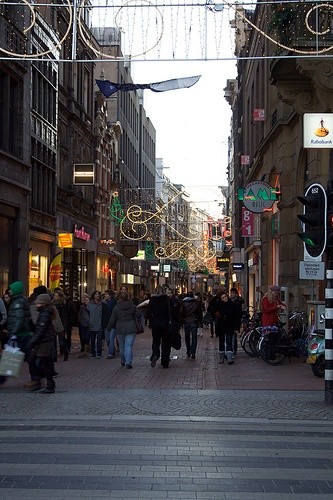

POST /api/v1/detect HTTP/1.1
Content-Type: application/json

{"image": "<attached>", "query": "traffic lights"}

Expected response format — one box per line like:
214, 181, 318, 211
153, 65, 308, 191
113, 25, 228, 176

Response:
296, 183, 328, 259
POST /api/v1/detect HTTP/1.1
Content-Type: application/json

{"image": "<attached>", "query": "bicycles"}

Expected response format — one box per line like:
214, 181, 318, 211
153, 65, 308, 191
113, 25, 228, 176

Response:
239, 305, 312, 366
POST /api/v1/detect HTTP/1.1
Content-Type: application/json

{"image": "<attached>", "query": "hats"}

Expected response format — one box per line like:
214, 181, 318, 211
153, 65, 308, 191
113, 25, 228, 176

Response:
270, 286, 280, 291
33, 293, 51, 305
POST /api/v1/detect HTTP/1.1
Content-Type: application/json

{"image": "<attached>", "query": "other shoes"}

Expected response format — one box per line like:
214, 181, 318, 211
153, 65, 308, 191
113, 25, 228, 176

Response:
39, 388, 56, 394
151, 356, 157, 368
96, 356, 100, 359
192, 355, 195, 359
126, 364, 132, 369
24, 380, 42, 388
53, 372, 57, 377
121, 362, 125, 366
64, 351, 68, 361
116, 347, 120, 352
163, 366, 169, 368
187, 354, 191, 357
107, 354, 113, 359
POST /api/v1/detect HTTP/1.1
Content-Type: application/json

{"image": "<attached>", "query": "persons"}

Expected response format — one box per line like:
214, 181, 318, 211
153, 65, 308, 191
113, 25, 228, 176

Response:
29, 293, 57, 393
30, 286, 74, 361
76, 290, 116, 359
168, 290, 180, 309
209, 291, 238, 365
147, 286, 173, 368
262, 286, 286, 361
230, 289, 245, 355
195, 291, 218, 337
180, 292, 203, 359
0, 280, 42, 389
107, 291, 141, 368
120, 286, 128, 292
133, 289, 154, 333
0, 289, 10, 329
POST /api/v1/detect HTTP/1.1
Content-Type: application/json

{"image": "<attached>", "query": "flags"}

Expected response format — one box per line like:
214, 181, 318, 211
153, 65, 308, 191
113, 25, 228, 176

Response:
47, 251, 62, 291
28, 247, 32, 278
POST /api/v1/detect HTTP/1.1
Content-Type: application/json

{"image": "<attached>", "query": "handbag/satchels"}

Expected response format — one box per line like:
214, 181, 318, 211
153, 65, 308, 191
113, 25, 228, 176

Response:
215, 311, 220, 318
0, 339, 25, 378
134, 308, 144, 334
167, 322, 176, 336
172, 331, 181, 350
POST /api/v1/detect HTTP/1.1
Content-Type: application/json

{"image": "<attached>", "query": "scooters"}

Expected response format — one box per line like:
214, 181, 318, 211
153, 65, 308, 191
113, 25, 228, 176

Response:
305, 328, 325, 378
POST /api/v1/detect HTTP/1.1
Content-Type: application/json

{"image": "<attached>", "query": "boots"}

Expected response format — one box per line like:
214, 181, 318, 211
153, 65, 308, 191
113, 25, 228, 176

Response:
226, 351, 234, 365
102, 339, 105, 349
197, 328, 204, 336
218, 351, 225, 363
